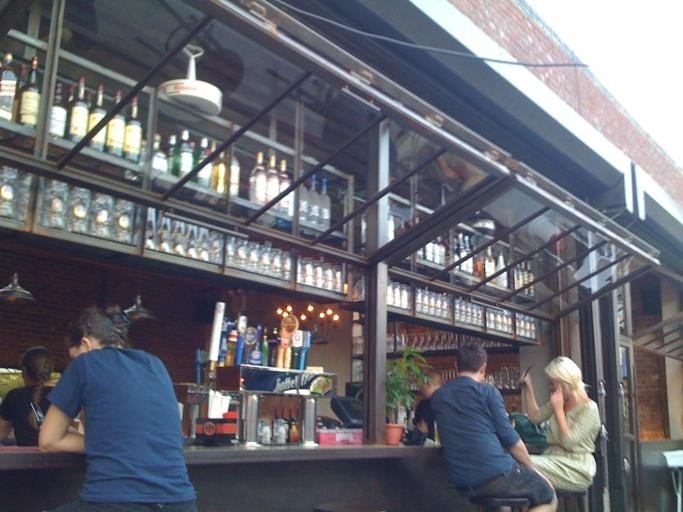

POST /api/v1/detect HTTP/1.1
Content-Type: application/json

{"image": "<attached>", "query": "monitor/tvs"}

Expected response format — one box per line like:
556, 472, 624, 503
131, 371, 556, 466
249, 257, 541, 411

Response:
331, 396, 366, 426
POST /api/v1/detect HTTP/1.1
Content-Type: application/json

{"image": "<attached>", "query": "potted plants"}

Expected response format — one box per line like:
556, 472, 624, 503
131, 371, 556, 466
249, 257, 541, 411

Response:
357, 346, 434, 445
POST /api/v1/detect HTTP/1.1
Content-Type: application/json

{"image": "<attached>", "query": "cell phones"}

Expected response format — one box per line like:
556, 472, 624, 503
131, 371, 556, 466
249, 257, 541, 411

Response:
30, 398, 45, 424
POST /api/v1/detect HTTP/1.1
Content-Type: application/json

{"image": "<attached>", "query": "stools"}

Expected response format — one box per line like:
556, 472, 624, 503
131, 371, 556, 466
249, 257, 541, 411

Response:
471, 497, 530, 512
555, 489, 590, 512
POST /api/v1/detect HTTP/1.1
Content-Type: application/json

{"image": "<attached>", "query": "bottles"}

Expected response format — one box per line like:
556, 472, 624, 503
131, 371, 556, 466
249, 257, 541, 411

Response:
219, 315, 310, 368
270, 406, 300, 443
388, 211, 533, 296
0, 45, 346, 291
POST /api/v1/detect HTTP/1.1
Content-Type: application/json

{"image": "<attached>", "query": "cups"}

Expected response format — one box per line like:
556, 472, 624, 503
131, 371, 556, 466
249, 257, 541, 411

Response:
235, 393, 258, 442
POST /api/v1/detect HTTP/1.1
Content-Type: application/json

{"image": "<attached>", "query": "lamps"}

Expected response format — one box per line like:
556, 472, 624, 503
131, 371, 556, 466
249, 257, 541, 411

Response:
123, 274, 160, 323
1, 252, 34, 304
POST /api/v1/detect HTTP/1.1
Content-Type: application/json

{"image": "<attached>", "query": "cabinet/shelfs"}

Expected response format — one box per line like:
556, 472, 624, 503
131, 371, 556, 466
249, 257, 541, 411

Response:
373, 188, 546, 346
0, 53, 355, 298
353, 312, 521, 396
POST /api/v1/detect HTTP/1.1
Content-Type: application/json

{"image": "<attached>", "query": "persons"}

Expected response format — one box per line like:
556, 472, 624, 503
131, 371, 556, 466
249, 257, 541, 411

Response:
413, 371, 446, 442
38, 305, 197, 510
516, 355, 600, 511
428, 342, 558, 511
0, 346, 56, 446
